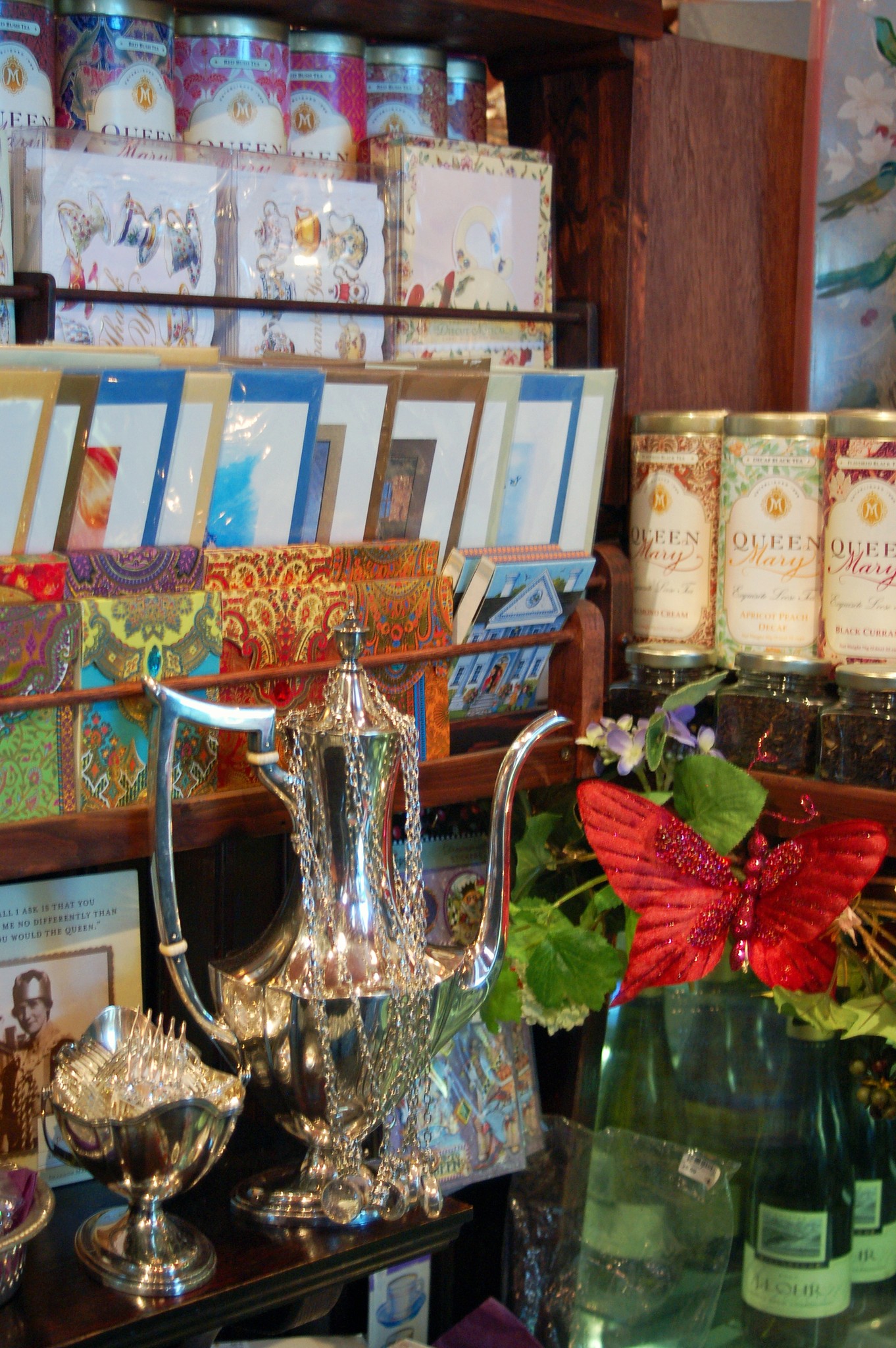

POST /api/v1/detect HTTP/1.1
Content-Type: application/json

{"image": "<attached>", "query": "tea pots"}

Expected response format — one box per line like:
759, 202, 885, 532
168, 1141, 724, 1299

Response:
137, 603, 572, 1229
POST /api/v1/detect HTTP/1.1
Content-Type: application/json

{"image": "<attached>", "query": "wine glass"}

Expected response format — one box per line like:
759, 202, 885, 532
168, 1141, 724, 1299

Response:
40, 1005, 248, 1297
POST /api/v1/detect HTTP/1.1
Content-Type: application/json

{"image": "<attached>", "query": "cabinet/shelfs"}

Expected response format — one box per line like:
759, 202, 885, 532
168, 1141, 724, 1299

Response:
0, 0, 896, 1348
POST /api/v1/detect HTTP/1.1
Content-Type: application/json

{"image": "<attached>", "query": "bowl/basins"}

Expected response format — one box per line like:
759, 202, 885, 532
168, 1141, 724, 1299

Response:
0, 1165, 55, 1308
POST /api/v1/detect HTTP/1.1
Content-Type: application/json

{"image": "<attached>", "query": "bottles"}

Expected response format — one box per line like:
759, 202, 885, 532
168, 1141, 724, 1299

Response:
818, 406, 895, 665
603, 642, 727, 762
364, 45, 449, 182
813, 665, 895, 794
712, 413, 830, 670
446, 58, 488, 145
0, 0, 55, 148
571, 982, 895, 1347
290, 31, 372, 184
630, 407, 726, 649
177, 13, 290, 158
718, 651, 833, 780
55, 0, 177, 161
